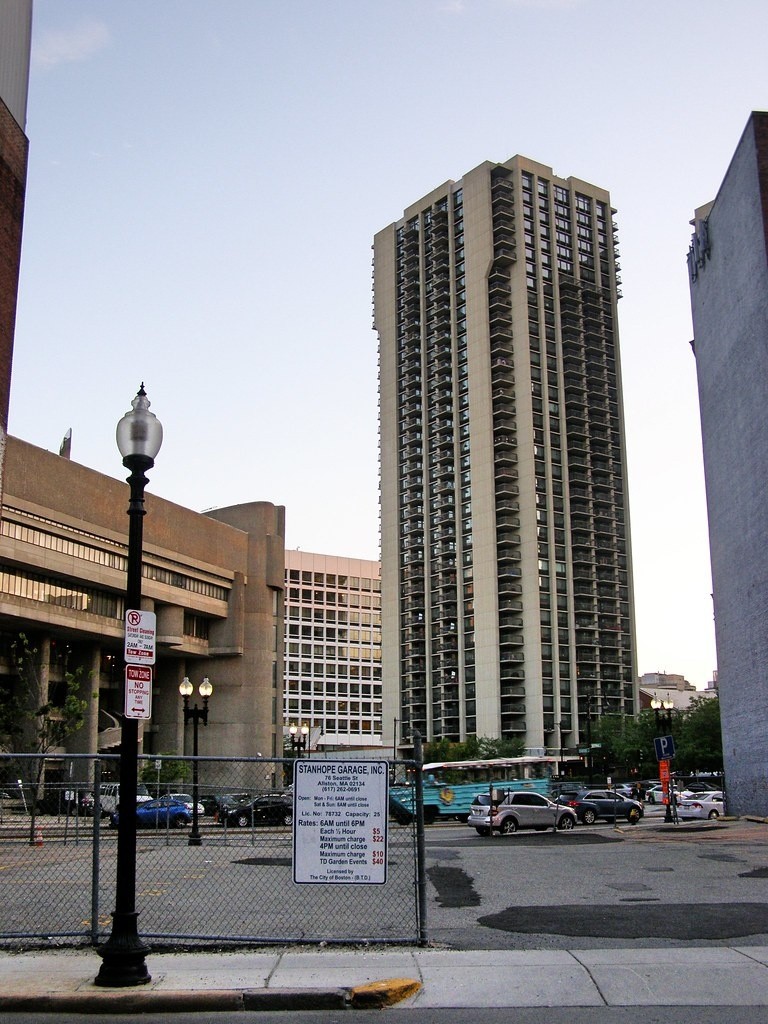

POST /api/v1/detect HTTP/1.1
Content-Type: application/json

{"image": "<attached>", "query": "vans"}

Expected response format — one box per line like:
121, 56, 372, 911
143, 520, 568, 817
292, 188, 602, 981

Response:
79, 782, 153, 819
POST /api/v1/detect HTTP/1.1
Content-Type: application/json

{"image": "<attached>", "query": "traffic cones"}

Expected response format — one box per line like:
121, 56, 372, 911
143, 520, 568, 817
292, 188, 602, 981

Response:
36, 826, 44, 847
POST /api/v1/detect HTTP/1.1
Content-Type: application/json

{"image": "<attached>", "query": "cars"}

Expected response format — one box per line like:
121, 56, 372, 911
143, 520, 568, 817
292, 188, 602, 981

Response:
609, 778, 722, 805
198, 795, 240, 816
670, 791, 726, 822
109, 798, 192, 829
218, 795, 294, 828
158, 793, 206, 819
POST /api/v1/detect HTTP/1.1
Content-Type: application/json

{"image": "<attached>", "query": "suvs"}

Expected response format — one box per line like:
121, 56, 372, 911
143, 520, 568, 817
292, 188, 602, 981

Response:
467, 789, 577, 837
553, 788, 644, 826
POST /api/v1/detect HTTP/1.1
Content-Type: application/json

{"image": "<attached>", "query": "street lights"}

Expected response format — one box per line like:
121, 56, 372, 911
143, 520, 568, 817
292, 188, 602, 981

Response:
649, 691, 676, 824
178, 676, 213, 846
585, 688, 611, 784
288, 721, 309, 759
91, 383, 162, 988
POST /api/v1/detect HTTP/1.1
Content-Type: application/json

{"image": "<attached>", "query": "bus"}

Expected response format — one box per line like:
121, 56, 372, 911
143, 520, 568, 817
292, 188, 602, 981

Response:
388, 756, 553, 826
